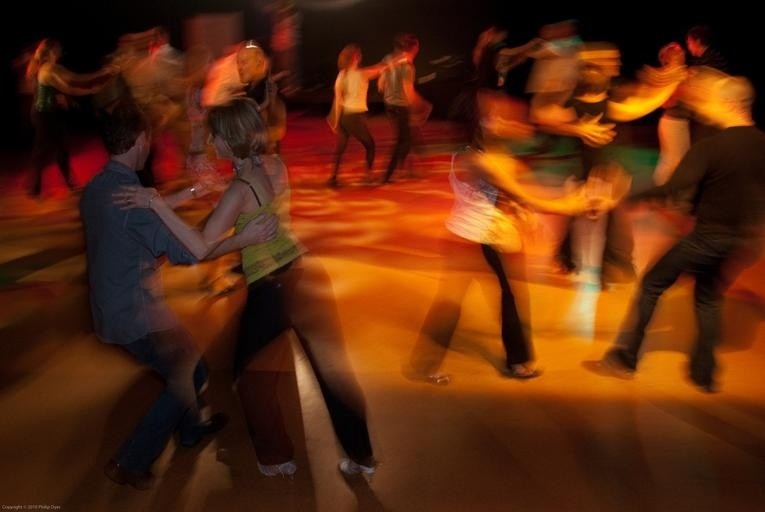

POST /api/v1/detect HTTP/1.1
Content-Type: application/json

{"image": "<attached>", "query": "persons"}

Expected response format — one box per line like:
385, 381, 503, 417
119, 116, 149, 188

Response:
20, 19, 765, 488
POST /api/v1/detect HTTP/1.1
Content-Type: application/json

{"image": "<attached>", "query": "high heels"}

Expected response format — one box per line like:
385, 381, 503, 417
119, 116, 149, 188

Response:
340, 461, 376, 483
257, 460, 297, 480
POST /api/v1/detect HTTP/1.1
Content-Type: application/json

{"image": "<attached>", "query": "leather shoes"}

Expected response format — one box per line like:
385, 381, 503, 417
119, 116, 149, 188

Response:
104, 459, 155, 491
